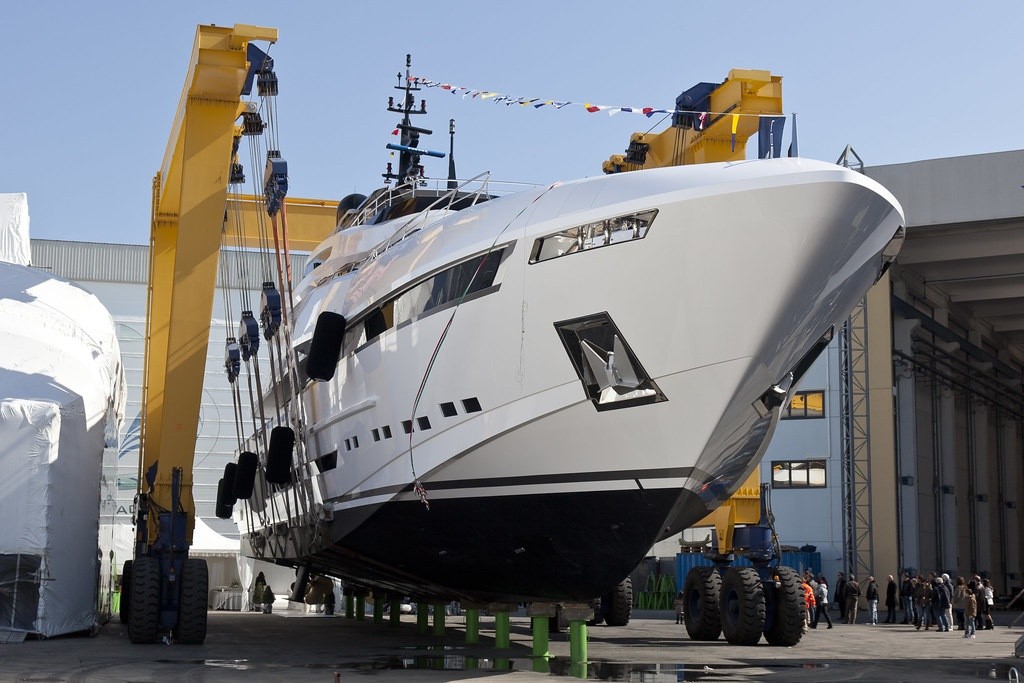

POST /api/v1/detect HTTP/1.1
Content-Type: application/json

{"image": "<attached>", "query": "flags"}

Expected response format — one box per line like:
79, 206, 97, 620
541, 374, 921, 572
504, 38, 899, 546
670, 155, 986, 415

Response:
408, 74, 741, 152
390, 152, 394, 158
391, 130, 398, 135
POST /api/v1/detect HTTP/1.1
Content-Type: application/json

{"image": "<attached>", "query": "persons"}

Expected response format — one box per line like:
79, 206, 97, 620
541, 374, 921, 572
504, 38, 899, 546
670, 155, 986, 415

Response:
884, 575, 898, 623
802, 571, 817, 633
842, 574, 860, 624
675, 592, 683, 624
808, 577, 833, 629
834, 573, 848, 620
901, 572, 995, 638
866, 576, 879, 625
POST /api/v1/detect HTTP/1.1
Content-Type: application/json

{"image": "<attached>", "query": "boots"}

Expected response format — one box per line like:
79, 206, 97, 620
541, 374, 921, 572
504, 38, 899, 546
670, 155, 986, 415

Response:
984, 620, 994, 630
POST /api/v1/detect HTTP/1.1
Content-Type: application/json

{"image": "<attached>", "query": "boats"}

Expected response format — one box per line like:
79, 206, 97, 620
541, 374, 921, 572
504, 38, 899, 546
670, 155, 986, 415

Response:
216, 50, 905, 649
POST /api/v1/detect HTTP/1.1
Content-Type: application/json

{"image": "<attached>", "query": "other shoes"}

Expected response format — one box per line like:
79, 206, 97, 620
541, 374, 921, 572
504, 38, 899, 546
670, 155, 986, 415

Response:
675, 622, 680, 624
963, 634, 975, 638
937, 629, 944, 632
866, 623, 876, 626
808, 624, 816, 629
944, 629, 949, 632
827, 624, 832, 629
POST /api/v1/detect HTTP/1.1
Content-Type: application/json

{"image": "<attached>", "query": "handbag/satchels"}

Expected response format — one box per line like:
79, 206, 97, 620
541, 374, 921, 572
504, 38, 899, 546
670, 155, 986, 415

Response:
816, 588, 823, 605
